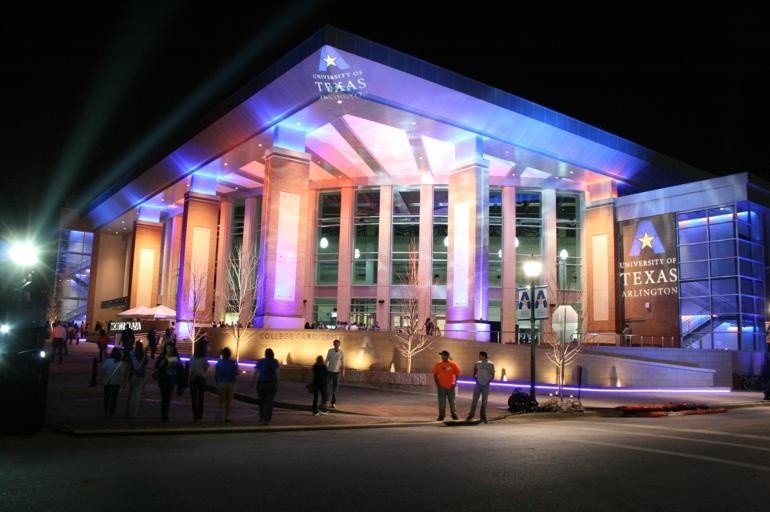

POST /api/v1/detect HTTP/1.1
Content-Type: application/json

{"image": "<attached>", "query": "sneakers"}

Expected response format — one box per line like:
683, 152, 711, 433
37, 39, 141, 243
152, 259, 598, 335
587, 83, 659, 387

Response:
312, 403, 335, 416
437, 413, 487, 423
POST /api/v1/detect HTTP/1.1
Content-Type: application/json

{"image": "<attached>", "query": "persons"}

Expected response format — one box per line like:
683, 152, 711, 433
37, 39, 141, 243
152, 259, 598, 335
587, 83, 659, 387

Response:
424, 316, 434, 336
431, 349, 461, 421
434, 326, 442, 337
214, 346, 238, 424
187, 342, 210, 424
312, 355, 331, 416
250, 348, 281, 425
465, 351, 495, 424
212, 319, 254, 328
518, 333, 539, 345
305, 319, 380, 331
622, 323, 632, 346
325, 340, 346, 409
44, 317, 187, 425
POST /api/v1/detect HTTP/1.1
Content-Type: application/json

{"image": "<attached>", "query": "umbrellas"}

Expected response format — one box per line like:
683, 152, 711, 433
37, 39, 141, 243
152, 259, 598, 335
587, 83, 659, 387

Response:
143, 304, 177, 322
116, 305, 155, 322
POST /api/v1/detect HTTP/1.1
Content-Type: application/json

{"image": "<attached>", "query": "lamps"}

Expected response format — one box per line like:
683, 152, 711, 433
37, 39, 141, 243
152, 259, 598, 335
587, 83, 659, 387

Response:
560, 249, 568, 260
516, 238, 519, 248
319, 237, 328, 248
443, 236, 448, 247
354, 249, 360, 259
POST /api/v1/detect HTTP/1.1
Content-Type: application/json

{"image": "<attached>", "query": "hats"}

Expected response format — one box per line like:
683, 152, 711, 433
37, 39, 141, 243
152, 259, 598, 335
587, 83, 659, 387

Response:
439, 351, 449, 355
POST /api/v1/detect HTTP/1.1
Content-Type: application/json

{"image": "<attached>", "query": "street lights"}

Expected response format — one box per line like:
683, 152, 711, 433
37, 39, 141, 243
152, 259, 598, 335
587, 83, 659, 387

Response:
523, 258, 544, 407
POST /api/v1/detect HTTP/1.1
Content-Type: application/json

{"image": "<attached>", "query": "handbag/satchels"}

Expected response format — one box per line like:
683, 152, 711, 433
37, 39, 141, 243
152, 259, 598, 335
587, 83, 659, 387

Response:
98, 369, 160, 385
